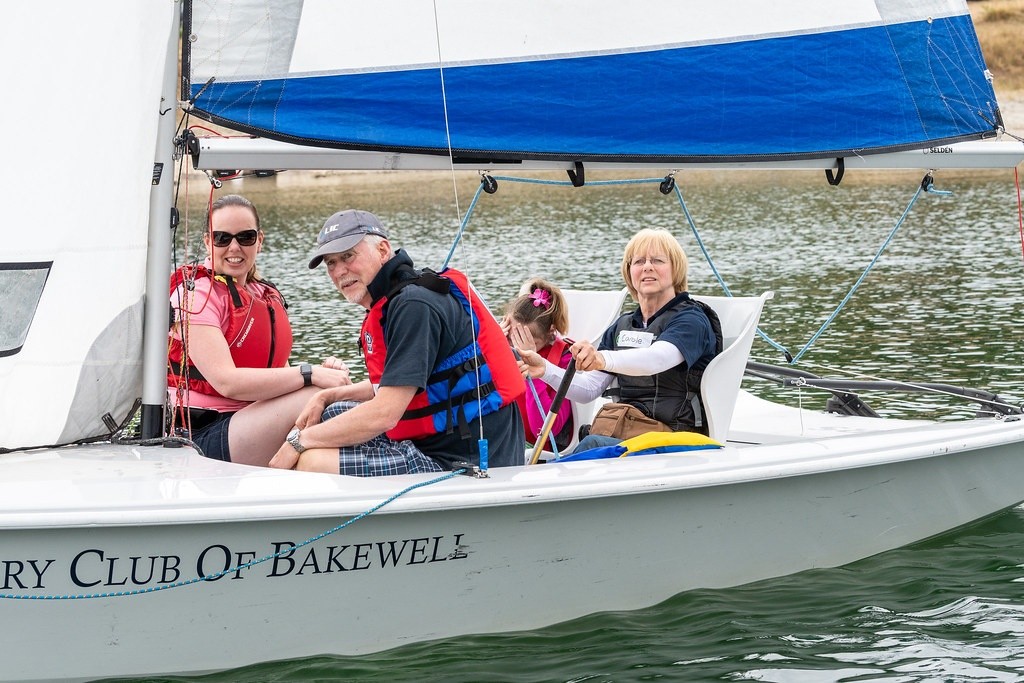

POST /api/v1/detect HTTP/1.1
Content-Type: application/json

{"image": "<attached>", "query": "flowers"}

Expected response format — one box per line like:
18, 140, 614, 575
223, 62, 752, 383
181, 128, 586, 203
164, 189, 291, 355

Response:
527, 289, 554, 310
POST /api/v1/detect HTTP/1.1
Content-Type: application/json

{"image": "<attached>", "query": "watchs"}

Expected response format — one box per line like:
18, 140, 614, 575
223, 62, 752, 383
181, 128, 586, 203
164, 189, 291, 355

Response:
300, 363, 313, 387
286, 428, 307, 454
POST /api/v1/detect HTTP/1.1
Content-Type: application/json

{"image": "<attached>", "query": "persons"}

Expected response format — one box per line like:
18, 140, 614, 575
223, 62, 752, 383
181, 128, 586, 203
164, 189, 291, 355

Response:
513, 227, 724, 466
496, 280, 575, 464
162, 195, 355, 468
268, 210, 527, 474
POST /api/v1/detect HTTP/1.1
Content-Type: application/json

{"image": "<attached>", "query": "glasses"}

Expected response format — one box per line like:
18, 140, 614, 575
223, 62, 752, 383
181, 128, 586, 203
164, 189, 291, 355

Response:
209, 229, 259, 247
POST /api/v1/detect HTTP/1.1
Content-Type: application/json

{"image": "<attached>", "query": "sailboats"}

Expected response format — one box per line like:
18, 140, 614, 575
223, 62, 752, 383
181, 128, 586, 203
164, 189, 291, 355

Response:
0, 1, 1024, 683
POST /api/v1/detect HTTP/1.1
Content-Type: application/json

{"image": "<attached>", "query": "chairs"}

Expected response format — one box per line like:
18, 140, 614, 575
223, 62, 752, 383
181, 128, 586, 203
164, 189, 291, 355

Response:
688, 290, 775, 448
518, 276, 631, 465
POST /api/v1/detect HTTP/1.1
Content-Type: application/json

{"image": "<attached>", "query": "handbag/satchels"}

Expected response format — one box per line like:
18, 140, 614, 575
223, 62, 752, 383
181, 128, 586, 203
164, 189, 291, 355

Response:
589, 403, 673, 440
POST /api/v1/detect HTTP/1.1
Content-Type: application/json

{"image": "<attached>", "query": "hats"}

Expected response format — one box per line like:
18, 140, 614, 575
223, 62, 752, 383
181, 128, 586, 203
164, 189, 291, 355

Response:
308, 209, 386, 270
